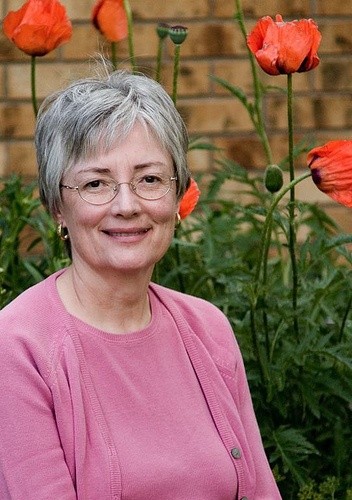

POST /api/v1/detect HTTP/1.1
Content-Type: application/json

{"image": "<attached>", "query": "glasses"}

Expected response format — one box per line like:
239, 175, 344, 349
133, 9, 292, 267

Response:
60, 171, 177, 205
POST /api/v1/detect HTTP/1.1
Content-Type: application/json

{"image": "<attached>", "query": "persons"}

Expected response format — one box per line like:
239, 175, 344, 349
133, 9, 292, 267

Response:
0, 73, 283, 500
